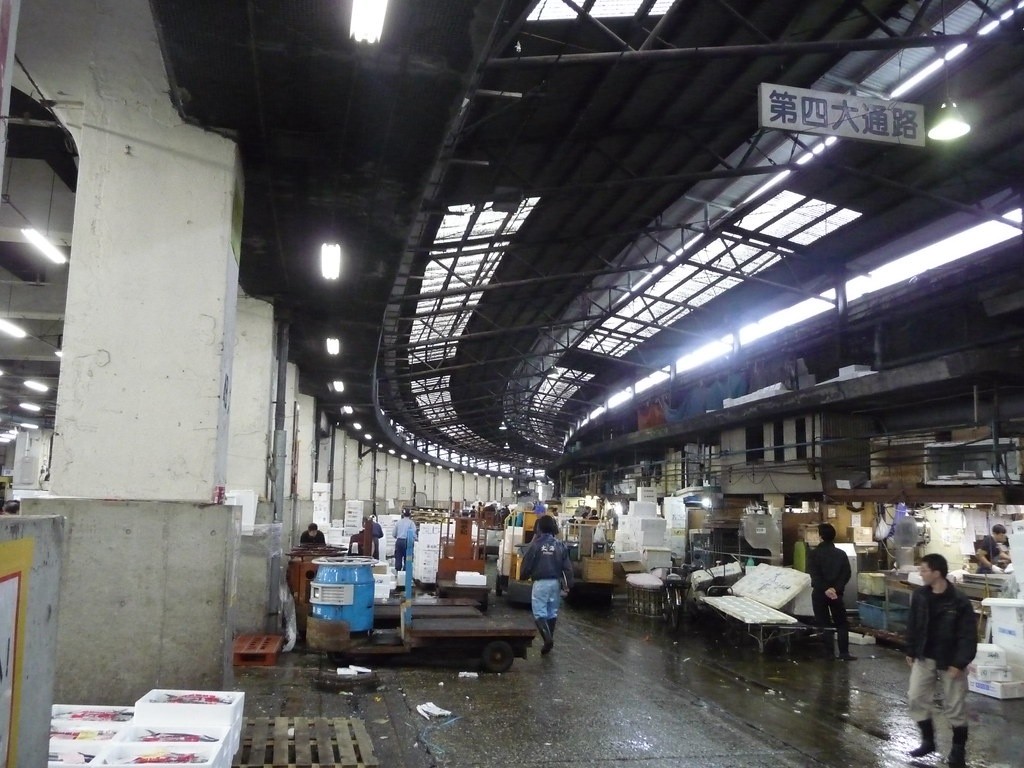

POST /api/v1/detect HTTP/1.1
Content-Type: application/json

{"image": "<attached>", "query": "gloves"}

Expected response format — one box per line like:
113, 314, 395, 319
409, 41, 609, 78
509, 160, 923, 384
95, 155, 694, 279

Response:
992, 564, 1004, 574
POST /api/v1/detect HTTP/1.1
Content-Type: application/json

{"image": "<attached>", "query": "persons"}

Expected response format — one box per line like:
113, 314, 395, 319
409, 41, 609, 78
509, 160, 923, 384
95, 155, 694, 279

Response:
975, 524, 1011, 574
516, 501, 599, 527
467, 502, 483, 518
394, 507, 418, 573
300, 523, 325, 545
905, 553, 977, 768
520, 515, 575, 655
808, 523, 858, 662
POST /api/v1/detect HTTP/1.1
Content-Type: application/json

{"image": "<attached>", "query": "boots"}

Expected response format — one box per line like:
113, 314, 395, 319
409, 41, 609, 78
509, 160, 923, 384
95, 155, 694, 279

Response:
839, 640, 857, 661
535, 617, 554, 655
910, 720, 935, 757
948, 726, 967, 768
547, 617, 556, 647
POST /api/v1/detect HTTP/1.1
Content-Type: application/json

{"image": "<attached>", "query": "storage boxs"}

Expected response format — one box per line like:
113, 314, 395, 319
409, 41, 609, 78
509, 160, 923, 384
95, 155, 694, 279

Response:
856, 601, 908, 631
839, 365, 871, 376
858, 572, 900, 595
847, 526, 873, 543
971, 643, 1006, 667
967, 675, 1024, 699
47, 689, 245, 768
313, 483, 707, 620
967, 665, 1011, 681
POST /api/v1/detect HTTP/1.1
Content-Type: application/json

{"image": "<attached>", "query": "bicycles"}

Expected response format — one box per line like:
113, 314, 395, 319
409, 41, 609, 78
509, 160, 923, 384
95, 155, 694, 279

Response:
649, 567, 690, 633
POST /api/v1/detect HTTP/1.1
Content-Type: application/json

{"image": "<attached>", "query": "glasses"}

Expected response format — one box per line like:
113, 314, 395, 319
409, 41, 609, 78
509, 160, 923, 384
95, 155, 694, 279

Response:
918, 568, 937, 574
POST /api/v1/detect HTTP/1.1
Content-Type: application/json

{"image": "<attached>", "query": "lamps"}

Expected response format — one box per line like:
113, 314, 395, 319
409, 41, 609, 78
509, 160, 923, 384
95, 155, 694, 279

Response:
927, 0, 971, 142
504, 432, 510, 449
499, 421, 507, 430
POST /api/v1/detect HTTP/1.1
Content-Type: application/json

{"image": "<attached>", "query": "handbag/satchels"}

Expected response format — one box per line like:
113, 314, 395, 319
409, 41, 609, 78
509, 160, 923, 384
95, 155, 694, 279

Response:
874, 515, 892, 540
